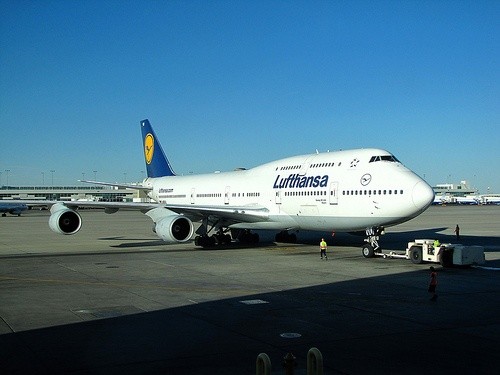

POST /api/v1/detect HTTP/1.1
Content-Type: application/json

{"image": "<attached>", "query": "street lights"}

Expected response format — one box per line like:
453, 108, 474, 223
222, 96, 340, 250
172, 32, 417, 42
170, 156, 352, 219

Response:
50, 170, 55, 190
5, 169, 10, 191
93, 170, 98, 190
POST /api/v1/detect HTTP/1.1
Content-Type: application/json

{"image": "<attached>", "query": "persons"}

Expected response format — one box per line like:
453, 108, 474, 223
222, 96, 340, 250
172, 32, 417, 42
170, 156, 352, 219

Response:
319, 238, 327, 259
454, 224, 460, 239
428, 266, 439, 301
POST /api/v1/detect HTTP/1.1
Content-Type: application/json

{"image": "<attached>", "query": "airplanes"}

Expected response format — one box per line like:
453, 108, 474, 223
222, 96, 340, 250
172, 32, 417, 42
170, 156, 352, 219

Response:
0, 118, 436, 258
